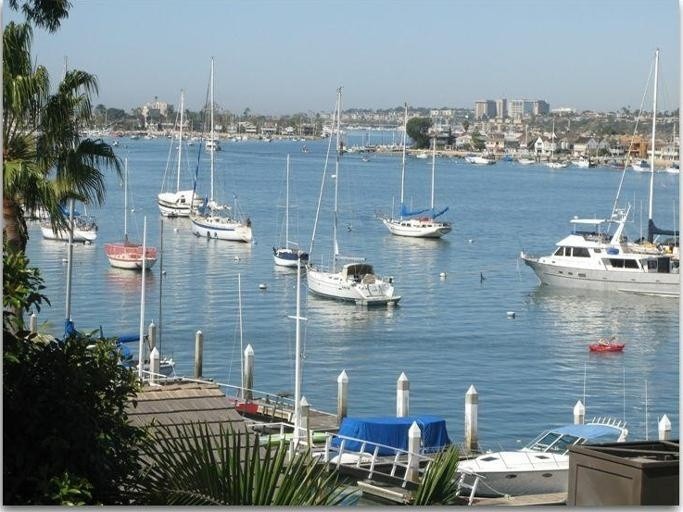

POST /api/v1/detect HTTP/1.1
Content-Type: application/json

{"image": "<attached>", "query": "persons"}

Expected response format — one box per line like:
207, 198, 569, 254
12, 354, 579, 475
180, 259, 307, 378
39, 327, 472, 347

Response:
655, 242, 664, 255
598, 338, 609, 347
672, 262, 680, 274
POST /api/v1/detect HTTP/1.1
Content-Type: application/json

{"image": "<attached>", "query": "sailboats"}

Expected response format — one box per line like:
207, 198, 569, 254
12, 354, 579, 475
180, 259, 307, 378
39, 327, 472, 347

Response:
55, 114, 172, 381
271, 153, 308, 269
79, 114, 305, 152
155, 86, 197, 219
519, 46, 680, 295
377, 102, 451, 239
102, 155, 156, 272
304, 85, 401, 307
187, 56, 255, 245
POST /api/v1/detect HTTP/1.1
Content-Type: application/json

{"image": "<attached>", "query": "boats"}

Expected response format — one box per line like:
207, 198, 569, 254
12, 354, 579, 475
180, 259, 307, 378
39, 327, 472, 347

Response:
452, 416, 631, 499
338, 113, 680, 175
587, 344, 625, 353
19, 202, 50, 220
37, 214, 97, 242
310, 413, 457, 473
